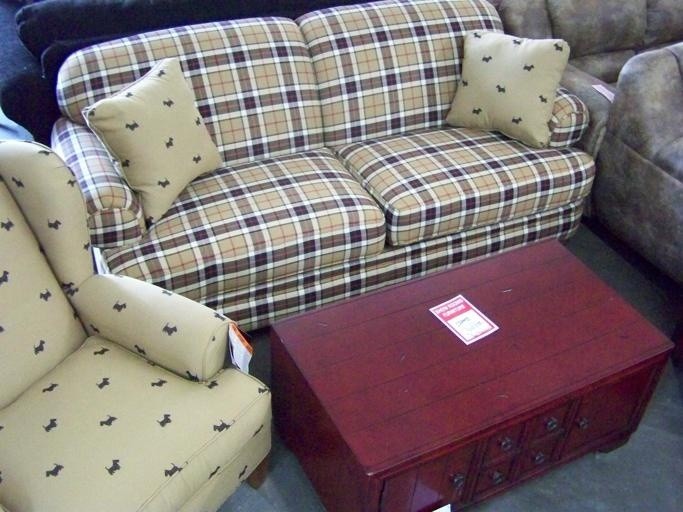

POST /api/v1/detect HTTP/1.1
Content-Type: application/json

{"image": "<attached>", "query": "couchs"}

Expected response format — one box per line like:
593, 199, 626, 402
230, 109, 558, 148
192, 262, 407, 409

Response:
51, 0, 597, 332
501, 0, 683, 219
595, 43, 682, 286
1, 0, 379, 145
1, 138, 273, 512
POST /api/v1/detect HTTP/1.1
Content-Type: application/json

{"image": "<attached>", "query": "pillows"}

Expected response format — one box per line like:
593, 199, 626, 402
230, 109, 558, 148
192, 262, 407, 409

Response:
81, 58, 225, 223
445, 29, 572, 149
